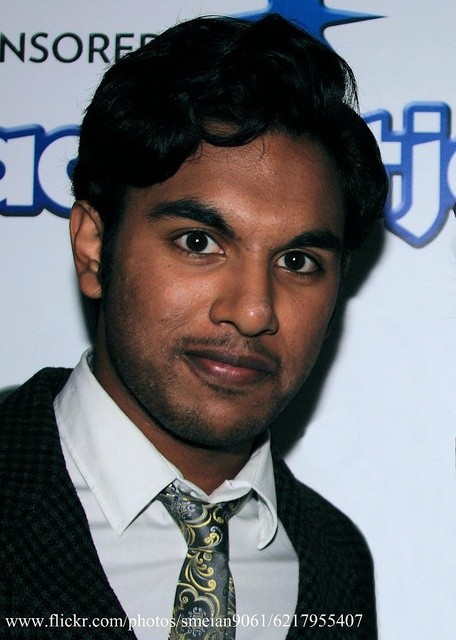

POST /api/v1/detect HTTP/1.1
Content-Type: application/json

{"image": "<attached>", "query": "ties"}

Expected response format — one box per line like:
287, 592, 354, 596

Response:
154, 484, 257, 640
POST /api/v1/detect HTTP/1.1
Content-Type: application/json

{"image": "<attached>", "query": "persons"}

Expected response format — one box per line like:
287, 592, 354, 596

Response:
0, 13, 389, 640
